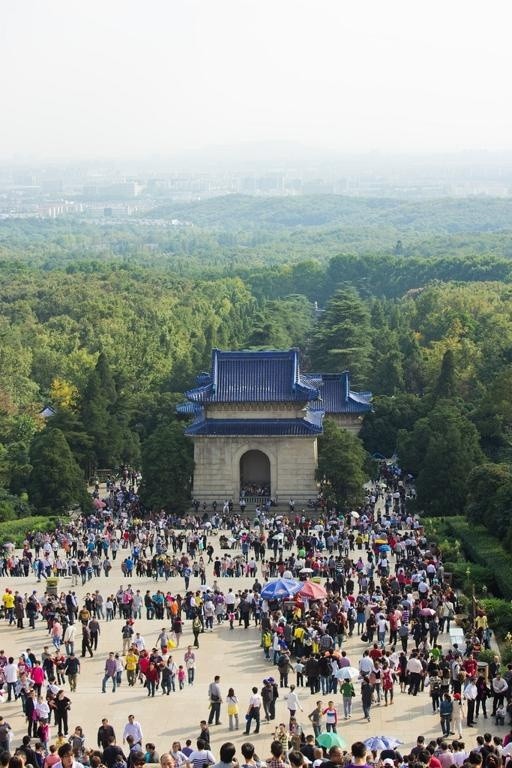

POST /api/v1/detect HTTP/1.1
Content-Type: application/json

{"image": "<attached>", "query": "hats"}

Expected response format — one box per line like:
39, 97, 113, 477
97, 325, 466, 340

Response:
268, 677, 274, 682
453, 693, 460, 700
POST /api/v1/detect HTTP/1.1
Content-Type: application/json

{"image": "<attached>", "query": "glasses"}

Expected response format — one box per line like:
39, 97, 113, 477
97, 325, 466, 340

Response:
134, 762, 145, 766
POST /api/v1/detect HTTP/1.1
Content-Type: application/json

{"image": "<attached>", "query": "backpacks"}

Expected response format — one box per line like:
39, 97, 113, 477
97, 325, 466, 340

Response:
382, 670, 393, 690
347, 608, 353, 619
372, 670, 381, 680
277, 655, 287, 668
431, 682, 441, 698
385, 623, 388, 632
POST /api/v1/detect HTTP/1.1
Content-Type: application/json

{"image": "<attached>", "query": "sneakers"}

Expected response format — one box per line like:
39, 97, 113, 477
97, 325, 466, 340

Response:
475, 712, 479, 718
491, 713, 505, 726
216, 721, 224, 725
23, 708, 69, 738
483, 712, 488, 719
228, 727, 241, 731
443, 732, 462, 740
400, 686, 424, 696
208, 719, 213, 724
265, 715, 270, 722
432, 709, 436, 715
344, 697, 395, 722
1, 615, 261, 635
470, 720, 477, 724
243, 732, 249, 735
252, 730, 260, 734
437, 706, 440, 712
270, 715, 276, 720
1, 638, 200, 703
467, 724, 473, 728
279, 684, 338, 695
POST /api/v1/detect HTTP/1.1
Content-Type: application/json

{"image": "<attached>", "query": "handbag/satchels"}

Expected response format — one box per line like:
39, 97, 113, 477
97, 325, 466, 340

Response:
351, 688, 356, 697
211, 695, 219, 702
360, 632, 369, 642
